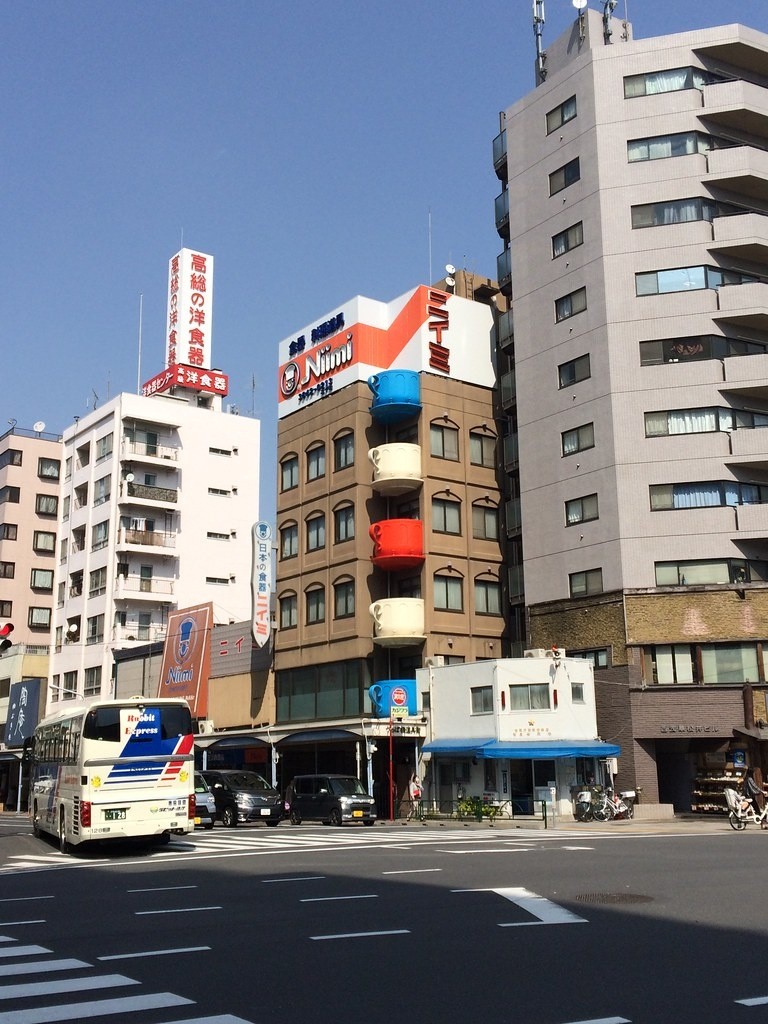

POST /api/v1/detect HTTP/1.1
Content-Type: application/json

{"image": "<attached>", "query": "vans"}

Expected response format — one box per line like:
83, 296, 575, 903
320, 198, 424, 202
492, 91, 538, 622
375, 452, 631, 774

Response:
195, 769, 285, 829
285, 772, 378, 827
194, 770, 216, 830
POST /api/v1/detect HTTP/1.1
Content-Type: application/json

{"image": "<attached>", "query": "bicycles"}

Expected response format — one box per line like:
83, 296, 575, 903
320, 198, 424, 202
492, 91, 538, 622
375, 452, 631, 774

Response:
577, 785, 635, 822
723, 785, 768, 831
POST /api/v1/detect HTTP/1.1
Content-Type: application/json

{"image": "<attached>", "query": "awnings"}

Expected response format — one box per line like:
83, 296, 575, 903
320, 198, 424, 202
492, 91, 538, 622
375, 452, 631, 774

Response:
421, 737, 498, 755
476, 741, 621, 761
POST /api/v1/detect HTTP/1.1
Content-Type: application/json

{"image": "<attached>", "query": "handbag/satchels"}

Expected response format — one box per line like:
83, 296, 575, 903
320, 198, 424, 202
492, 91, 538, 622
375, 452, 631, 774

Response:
414, 790, 419, 797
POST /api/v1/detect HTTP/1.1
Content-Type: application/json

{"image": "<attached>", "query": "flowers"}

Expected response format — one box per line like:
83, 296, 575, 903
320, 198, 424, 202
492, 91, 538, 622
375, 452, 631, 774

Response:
459, 797, 495, 820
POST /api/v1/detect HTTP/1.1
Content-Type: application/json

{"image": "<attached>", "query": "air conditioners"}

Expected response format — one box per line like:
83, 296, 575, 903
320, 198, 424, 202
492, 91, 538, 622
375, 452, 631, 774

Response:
523, 648, 544, 658
425, 656, 444, 668
198, 720, 214, 735
545, 648, 566, 658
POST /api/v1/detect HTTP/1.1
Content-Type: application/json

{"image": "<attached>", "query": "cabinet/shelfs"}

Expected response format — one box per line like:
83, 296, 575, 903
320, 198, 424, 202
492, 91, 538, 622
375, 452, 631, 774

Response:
690, 769, 749, 814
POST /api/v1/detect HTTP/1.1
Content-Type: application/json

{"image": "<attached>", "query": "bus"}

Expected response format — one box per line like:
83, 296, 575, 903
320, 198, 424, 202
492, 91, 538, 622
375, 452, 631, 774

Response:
21, 695, 197, 853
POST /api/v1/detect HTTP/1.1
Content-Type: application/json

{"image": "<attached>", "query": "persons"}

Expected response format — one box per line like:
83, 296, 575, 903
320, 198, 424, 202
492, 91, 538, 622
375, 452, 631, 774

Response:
723, 780, 750, 820
741, 767, 767, 830
405, 775, 425, 822
